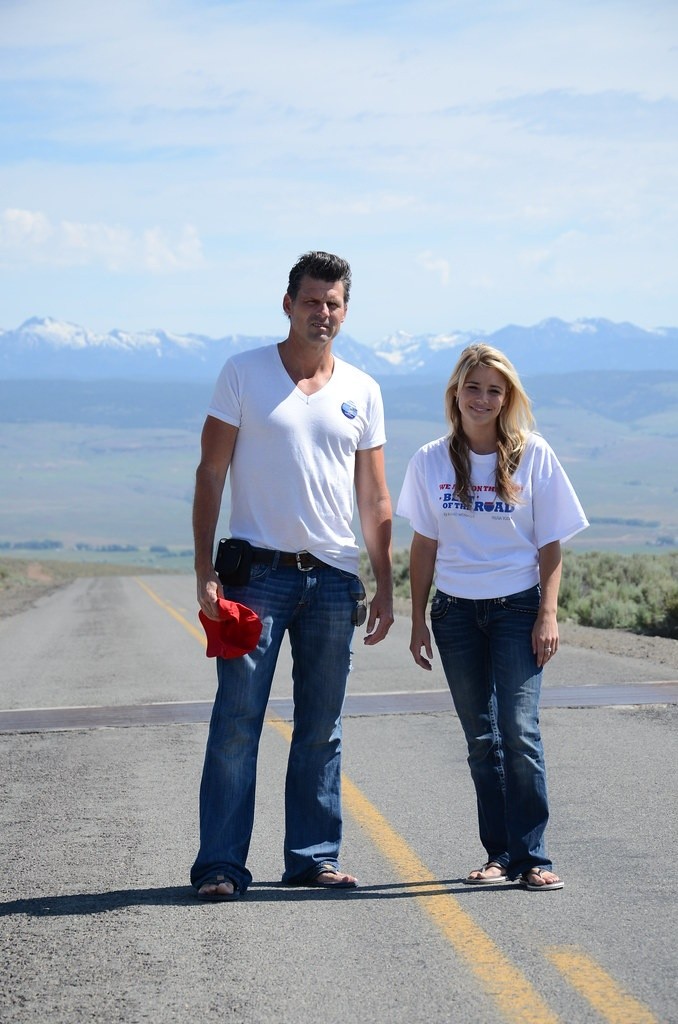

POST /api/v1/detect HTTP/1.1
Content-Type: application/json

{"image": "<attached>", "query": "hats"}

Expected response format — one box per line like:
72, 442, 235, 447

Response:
199, 599, 263, 659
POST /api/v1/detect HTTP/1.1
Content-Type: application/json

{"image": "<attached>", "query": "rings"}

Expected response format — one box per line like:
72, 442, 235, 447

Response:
545, 648, 551, 652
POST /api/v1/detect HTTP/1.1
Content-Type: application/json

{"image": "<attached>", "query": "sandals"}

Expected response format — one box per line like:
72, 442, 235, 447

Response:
282, 864, 357, 888
197, 874, 239, 901
354, 900, 361, 903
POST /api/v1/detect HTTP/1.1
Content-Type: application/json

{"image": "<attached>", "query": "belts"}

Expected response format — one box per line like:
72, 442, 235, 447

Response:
253, 549, 327, 572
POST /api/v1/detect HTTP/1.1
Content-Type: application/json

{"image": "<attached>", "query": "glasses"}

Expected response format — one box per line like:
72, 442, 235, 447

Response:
349, 576, 367, 627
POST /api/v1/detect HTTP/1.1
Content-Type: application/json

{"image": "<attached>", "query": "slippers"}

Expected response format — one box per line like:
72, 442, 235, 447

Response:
519, 868, 564, 890
466, 862, 521, 883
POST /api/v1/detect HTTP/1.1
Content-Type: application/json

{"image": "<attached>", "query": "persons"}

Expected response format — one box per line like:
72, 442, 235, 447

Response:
191, 252, 396, 902
397, 344, 590, 891
